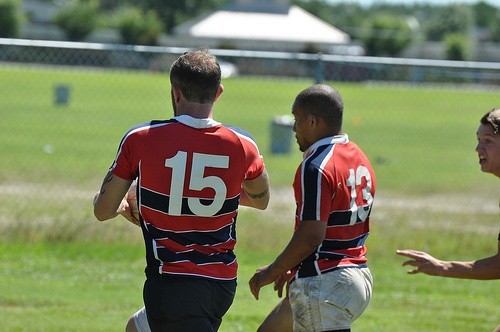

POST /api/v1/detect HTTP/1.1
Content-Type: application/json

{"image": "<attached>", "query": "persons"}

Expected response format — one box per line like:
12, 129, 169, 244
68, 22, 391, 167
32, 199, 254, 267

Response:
93, 48, 270, 332
250, 83, 374, 332
397, 108, 499, 280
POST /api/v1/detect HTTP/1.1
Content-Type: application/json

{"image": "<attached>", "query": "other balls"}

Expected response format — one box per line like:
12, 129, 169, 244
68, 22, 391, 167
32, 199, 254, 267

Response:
126, 178, 140, 224
